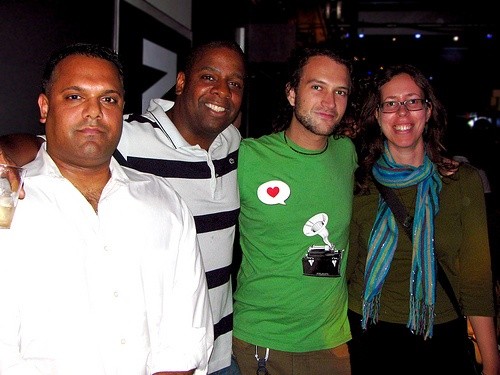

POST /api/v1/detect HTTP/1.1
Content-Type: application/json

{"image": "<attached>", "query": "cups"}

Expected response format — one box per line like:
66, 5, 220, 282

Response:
0, 163, 26, 230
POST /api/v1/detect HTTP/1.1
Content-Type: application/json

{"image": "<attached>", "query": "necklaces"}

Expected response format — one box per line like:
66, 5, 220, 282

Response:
282, 127, 329, 156
397, 189, 416, 227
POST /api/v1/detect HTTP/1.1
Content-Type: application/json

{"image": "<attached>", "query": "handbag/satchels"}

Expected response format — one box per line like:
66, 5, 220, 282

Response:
464, 313, 500, 365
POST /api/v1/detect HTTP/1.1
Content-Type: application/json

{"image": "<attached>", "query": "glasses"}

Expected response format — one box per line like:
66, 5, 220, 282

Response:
377, 99, 429, 113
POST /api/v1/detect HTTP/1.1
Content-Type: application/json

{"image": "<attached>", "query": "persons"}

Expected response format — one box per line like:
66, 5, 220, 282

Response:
346, 63, 500, 375
232, 46, 357, 375
0, 31, 244, 375
0, 42, 214, 375
